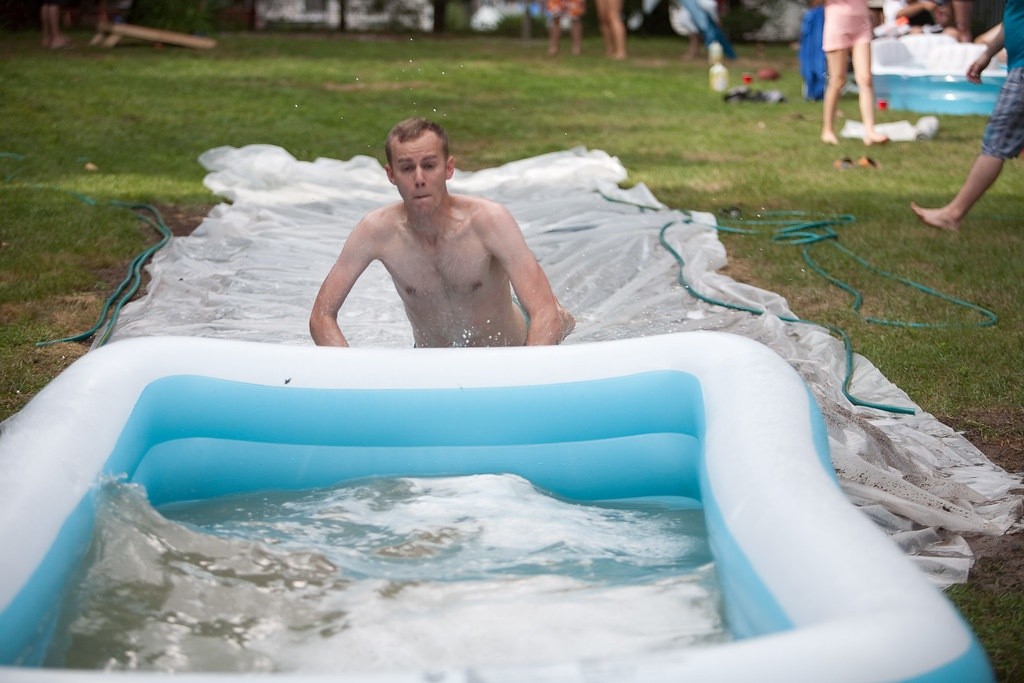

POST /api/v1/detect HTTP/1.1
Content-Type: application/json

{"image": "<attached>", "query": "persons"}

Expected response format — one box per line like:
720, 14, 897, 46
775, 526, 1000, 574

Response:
818, 0, 1008, 147
679, 0, 724, 61
545, 0, 587, 56
909, 0, 1024, 233
308, 116, 575, 348
39, 0, 132, 50
595, 0, 630, 60
